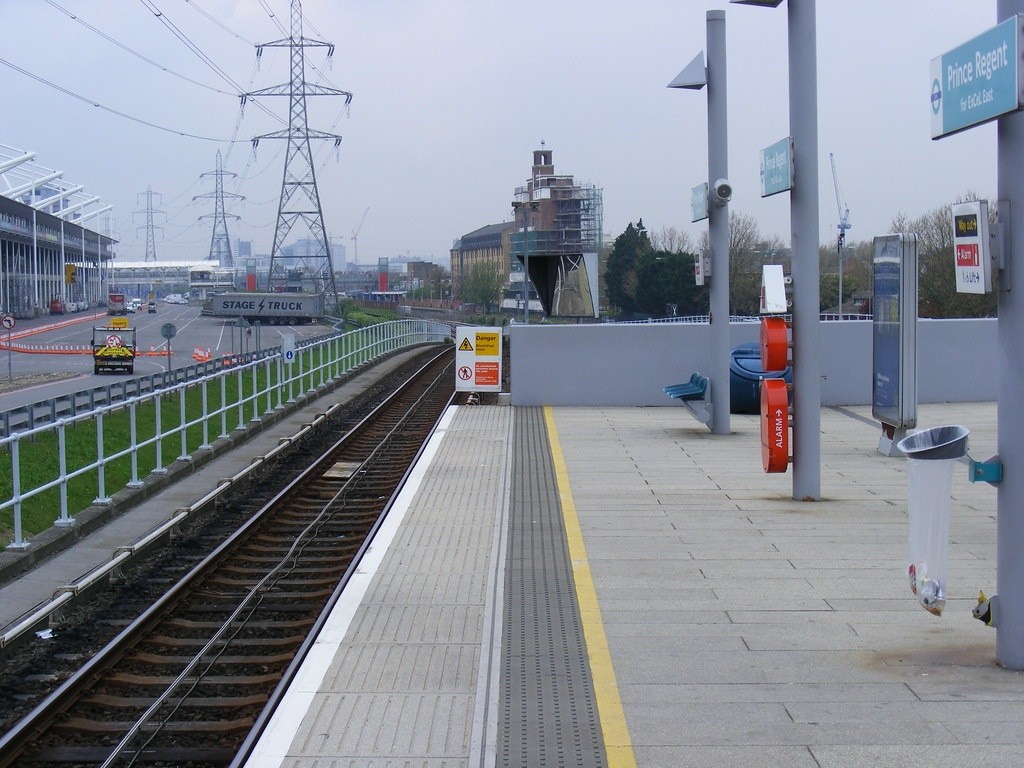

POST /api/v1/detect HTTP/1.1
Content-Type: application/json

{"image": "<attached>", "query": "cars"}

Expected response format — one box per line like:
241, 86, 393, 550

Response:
164, 292, 191, 305
126, 301, 137, 314
148, 300, 158, 313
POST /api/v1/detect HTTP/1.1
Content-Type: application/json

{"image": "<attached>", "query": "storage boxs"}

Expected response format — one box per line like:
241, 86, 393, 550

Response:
730, 343, 794, 414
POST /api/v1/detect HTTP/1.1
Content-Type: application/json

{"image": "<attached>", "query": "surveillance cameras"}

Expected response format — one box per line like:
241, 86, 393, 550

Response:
714, 178, 732, 200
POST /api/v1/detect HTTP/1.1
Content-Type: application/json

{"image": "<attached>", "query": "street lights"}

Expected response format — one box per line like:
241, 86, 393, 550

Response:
512, 200, 542, 325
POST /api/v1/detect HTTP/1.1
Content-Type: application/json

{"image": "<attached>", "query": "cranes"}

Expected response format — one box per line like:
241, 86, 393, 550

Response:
829, 151, 854, 249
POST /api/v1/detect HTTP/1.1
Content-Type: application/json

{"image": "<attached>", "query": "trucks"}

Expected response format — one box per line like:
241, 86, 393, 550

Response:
91, 322, 136, 375
109, 293, 127, 315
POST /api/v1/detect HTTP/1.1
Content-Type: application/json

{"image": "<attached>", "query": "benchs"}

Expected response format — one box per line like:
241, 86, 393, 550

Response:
663, 372, 709, 401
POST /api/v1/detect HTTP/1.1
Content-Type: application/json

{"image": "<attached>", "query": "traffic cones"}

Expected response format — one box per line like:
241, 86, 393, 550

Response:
133, 343, 237, 367
0, 312, 107, 356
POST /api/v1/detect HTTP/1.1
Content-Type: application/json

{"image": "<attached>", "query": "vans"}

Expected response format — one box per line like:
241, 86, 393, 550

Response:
133, 298, 144, 309
